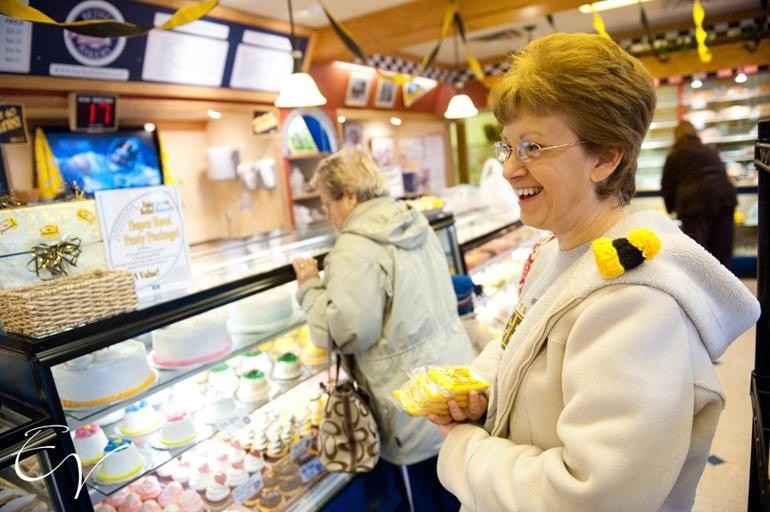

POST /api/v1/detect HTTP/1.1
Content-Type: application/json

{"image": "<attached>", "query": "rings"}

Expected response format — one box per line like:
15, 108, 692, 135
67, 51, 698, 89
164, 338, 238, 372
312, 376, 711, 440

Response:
299, 264, 304, 268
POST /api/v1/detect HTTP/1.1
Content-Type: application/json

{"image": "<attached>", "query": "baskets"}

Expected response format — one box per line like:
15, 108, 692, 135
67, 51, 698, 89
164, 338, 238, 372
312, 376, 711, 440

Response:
0, 269, 139, 340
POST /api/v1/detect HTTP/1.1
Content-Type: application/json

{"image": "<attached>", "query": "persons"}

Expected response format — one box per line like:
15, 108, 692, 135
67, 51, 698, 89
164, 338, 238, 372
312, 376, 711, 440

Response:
288, 146, 474, 511
414, 32, 764, 510
659, 119, 740, 272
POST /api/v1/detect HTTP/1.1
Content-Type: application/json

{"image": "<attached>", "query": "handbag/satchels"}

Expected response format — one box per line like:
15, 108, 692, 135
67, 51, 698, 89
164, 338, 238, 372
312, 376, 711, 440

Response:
317, 330, 381, 473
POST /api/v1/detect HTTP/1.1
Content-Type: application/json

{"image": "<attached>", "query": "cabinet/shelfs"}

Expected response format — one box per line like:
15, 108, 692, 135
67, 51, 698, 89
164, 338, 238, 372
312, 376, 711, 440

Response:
0, 69, 770, 512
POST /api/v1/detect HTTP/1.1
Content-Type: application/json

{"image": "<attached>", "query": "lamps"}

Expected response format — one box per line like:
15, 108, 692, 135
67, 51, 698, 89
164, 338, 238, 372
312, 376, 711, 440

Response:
274, 1, 327, 108
444, 1, 478, 120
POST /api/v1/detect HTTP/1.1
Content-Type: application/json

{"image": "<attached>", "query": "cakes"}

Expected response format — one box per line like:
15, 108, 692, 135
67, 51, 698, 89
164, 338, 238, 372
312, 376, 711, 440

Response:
50, 285, 330, 512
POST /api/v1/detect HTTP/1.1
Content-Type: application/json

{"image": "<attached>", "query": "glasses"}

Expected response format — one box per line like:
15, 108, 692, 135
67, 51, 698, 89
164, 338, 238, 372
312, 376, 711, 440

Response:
320, 204, 329, 213
494, 140, 588, 163
